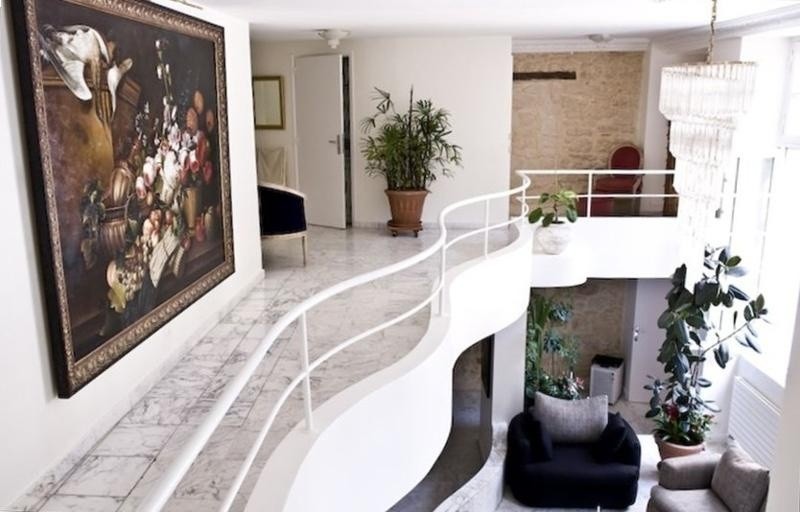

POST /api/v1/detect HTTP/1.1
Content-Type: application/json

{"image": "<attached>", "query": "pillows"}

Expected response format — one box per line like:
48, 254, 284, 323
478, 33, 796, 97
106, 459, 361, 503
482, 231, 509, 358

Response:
535, 392, 608, 443
711, 435, 769, 512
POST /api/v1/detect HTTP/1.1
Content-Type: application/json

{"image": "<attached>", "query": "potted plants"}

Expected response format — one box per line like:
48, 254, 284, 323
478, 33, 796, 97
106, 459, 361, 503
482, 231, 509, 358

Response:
643, 244, 772, 461
359, 86, 464, 238
528, 187, 579, 255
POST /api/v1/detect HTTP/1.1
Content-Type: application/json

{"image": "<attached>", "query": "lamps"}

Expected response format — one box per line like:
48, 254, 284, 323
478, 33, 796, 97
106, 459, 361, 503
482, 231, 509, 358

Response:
320, 30, 348, 48
574, 281, 593, 288
659, 62, 759, 240
590, 35, 612, 42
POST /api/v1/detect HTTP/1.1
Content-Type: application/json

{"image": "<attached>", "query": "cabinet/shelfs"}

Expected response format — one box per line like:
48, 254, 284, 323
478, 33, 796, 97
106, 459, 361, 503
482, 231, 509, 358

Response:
589, 362, 624, 405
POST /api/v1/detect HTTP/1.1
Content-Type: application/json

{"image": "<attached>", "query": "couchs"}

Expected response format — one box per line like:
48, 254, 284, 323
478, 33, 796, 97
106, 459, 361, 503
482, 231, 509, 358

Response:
647, 454, 733, 512
505, 412, 641, 509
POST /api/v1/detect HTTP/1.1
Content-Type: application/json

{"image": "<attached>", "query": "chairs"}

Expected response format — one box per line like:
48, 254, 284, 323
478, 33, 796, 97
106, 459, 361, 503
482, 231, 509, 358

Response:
258, 182, 308, 268
593, 142, 644, 216
576, 193, 613, 217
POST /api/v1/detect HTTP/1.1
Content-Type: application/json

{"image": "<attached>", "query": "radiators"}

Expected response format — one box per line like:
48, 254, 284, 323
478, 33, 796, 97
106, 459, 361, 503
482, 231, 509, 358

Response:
728, 376, 781, 470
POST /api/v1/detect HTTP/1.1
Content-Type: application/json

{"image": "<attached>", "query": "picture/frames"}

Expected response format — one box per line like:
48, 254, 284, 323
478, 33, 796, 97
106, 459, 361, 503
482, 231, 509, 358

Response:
481, 336, 493, 397
10, 0, 237, 399
252, 76, 286, 129
